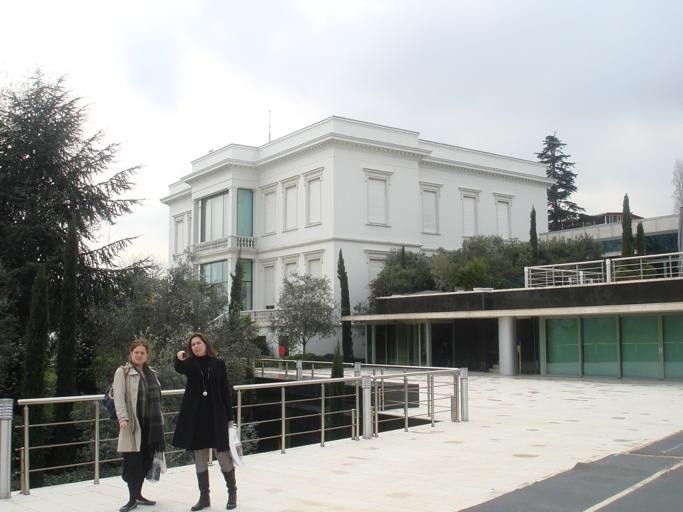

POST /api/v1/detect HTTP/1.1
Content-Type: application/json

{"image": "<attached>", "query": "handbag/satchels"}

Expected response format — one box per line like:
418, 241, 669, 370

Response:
146, 451, 167, 481
102, 384, 119, 421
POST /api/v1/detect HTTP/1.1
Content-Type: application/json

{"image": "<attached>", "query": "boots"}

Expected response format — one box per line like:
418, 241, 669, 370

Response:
191, 469, 210, 511
223, 467, 237, 509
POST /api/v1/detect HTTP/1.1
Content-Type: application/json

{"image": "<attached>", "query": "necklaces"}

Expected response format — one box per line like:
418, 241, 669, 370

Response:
198, 359, 211, 397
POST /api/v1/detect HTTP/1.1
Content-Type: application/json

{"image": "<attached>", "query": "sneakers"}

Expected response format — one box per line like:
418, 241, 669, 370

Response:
119, 498, 156, 512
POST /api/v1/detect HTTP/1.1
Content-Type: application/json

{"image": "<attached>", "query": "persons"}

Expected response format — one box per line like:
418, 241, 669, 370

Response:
277, 341, 287, 369
170, 333, 237, 511
114, 339, 166, 512
